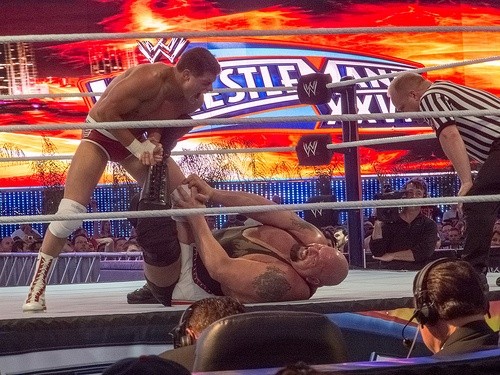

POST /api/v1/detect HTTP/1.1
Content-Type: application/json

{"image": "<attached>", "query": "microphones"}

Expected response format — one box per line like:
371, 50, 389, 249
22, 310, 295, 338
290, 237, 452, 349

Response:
400, 311, 419, 349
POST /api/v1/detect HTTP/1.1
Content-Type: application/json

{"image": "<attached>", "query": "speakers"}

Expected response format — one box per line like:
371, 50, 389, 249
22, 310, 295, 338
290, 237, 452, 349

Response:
41, 188, 63, 216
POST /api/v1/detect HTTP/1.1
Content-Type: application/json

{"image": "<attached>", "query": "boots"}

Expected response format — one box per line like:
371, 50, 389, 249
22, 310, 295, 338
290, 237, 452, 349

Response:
127, 283, 161, 304
22, 246, 59, 313
171, 238, 216, 303
141, 113, 194, 206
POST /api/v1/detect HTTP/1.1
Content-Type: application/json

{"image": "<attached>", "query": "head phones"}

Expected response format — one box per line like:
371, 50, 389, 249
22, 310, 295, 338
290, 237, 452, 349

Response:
414, 255, 490, 324
170, 295, 213, 350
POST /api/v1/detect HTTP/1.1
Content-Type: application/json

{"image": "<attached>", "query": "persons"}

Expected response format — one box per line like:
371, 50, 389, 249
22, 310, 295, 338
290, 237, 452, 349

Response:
0, 47, 500, 375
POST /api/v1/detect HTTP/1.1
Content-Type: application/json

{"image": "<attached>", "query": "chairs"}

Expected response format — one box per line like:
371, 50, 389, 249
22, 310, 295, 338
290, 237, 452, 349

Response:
192, 311, 352, 373
